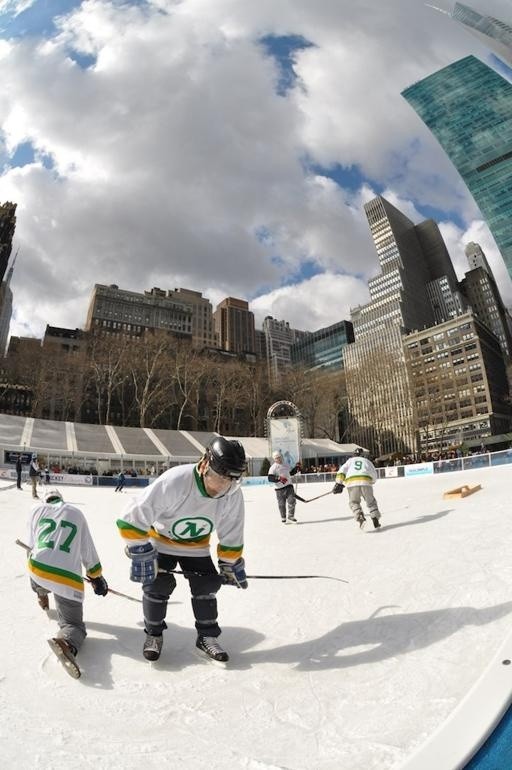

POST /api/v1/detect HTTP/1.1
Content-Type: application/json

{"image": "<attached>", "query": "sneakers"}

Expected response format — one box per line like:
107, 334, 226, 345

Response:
197, 635, 228, 661
143, 630, 163, 660
48, 636, 81, 679
359, 515, 366, 528
373, 518, 381, 527
288, 516, 298, 521
282, 517, 286, 522
38, 593, 49, 610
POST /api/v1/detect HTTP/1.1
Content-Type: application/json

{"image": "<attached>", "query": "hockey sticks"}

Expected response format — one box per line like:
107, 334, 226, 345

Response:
158, 568, 350, 586
295, 490, 333, 503
15, 539, 184, 604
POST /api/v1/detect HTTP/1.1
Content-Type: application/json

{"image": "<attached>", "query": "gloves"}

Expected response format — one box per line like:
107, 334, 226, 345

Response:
296, 463, 301, 470
218, 559, 247, 588
128, 543, 157, 583
90, 576, 108, 596
334, 483, 343, 493
279, 477, 287, 483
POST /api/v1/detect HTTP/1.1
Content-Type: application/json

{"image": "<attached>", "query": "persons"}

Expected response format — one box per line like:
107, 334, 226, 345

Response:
28, 489, 109, 678
288, 450, 465, 473
9, 454, 155, 495
267, 450, 298, 524
116, 436, 246, 661
333, 448, 382, 529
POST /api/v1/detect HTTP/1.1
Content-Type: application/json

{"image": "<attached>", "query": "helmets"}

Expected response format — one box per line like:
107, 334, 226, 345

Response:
273, 452, 283, 461
354, 448, 365, 456
207, 437, 245, 495
